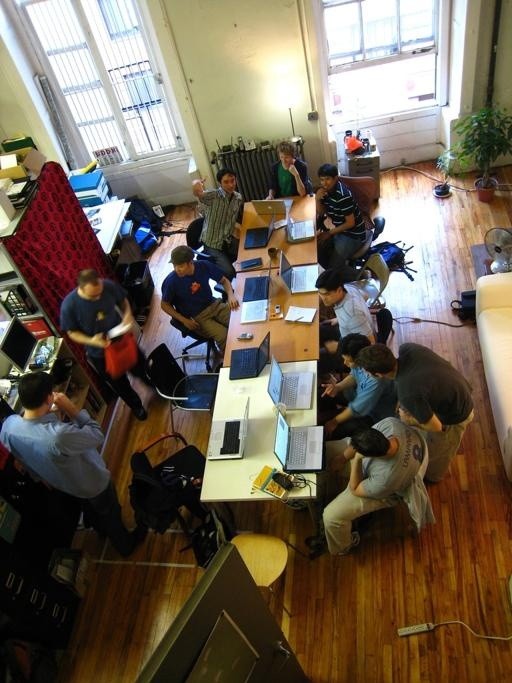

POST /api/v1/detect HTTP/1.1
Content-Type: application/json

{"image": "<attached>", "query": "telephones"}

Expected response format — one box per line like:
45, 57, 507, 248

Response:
237, 135, 257, 151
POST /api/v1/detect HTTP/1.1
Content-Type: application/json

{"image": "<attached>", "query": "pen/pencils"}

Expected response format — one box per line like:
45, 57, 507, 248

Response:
295, 316, 305, 322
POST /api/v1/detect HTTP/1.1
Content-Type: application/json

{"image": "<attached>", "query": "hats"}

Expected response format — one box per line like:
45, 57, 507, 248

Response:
168, 246, 194, 264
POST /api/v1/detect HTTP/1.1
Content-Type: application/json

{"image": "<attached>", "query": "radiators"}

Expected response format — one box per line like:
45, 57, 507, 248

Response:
216, 137, 307, 204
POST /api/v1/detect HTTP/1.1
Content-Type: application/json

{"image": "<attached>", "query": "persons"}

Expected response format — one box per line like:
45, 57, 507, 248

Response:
264, 141, 313, 200
354, 342, 475, 482
323, 417, 430, 556
160, 245, 240, 354
314, 164, 367, 265
317, 333, 397, 452
1, 372, 149, 556
315, 270, 381, 354
191, 168, 245, 281
59, 269, 157, 420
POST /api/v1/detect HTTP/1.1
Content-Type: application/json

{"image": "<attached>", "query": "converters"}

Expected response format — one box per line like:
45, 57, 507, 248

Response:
273, 473, 291, 488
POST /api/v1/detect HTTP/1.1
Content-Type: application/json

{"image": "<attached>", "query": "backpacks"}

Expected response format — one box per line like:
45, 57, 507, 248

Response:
130, 198, 163, 229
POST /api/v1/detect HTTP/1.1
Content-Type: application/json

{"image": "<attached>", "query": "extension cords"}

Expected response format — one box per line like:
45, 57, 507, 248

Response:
397, 622, 434, 636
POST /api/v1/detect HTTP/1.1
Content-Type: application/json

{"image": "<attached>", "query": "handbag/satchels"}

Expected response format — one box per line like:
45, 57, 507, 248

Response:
189, 510, 226, 567
134, 220, 158, 254
103, 323, 138, 379
143, 465, 201, 513
451, 290, 476, 321
358, 241, 417, 281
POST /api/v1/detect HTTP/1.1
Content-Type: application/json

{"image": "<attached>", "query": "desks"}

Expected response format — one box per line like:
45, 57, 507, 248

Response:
82, 203, 132, 265
197, 194, 323, 543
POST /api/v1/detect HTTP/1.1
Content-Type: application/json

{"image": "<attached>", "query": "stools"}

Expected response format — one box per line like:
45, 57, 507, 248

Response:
226, 533, 311, 619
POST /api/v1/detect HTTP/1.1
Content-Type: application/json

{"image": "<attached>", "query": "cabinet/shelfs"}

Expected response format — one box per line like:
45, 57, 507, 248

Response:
334, 126, 382, 205
9, 335, 132, 514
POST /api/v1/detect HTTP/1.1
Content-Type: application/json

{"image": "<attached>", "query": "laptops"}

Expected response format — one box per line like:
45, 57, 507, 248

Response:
230, 331, 270, 379
267, 355, 314, 410
243, 219, 274, 249
207, 397, 249, 460
278, 250, 319, 293
284, 217, 317, 243
273, 411, 325, 472
251, 199, 296, 217
239, 261, 271, 299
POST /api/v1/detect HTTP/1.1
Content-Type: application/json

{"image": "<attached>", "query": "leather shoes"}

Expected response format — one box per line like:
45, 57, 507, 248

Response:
121, 525, 148, 556
132, 407, 145, 419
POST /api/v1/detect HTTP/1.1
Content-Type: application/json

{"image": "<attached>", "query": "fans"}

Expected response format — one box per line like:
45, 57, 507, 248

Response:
485, 227, 512, 273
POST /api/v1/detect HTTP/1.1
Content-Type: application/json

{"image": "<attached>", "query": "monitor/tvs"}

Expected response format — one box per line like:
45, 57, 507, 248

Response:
1, 316, 37, 374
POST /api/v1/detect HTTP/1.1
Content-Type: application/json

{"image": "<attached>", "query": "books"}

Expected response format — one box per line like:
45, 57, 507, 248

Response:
120, 220, 133, 237
81, 389, 105, 420
7, 178, 38, 209
240, 257, 264, 271
252, 464, 296, 500
0, 284, 54, 340
68, 170, 110, 209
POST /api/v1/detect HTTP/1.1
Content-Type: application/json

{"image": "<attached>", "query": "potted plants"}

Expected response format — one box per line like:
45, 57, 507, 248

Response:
436, 103, 512, 202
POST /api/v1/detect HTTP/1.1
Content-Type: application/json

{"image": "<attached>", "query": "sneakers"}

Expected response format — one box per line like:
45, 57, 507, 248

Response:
338, 532, 360, 556
288, 499, 307, 510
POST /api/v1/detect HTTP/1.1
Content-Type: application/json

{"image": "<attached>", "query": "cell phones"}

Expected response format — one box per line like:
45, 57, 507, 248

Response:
275, 305, 280, 314
238, 334, 253, 339
